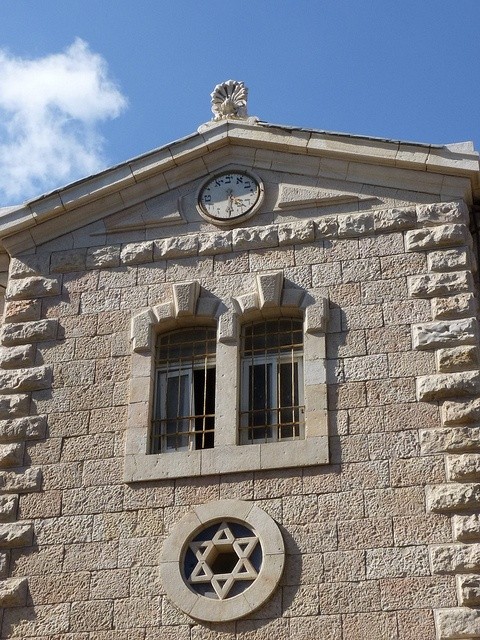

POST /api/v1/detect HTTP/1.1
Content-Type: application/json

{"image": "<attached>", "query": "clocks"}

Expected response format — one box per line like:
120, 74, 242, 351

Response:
195, 165, 265, 228
